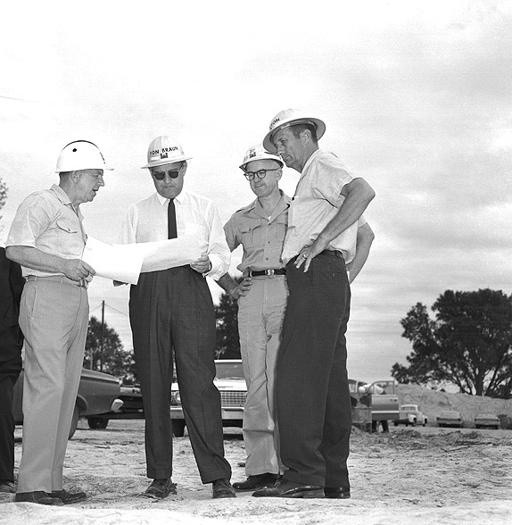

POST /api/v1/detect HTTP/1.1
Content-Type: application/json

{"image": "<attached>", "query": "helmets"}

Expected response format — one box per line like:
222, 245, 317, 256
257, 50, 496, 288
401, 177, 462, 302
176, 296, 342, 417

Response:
139, 134, 192, 170
54, 140, 115, 174
239, 144, 284, 172
263, 108, 326, 154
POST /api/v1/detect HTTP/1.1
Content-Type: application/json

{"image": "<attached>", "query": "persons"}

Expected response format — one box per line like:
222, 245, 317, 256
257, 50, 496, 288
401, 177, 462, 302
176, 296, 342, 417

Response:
215, 145, 296, 490
111, 134, 236, 498
252, 107, 375, 500
5, 140, 113, 507
358, 384, 390, 434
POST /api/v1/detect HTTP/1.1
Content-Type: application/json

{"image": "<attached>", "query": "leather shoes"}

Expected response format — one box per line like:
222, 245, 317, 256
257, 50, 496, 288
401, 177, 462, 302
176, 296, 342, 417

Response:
211, 479, 237, 498
324, 482, 350, 499
16, 492, 64, 507
252, 477, 326, 500
46, 491, 86, 504
144, 477, 173, 500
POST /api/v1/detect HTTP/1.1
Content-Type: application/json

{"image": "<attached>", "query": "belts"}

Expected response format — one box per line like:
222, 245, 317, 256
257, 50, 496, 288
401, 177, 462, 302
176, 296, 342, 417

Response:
246, 269, 287, 277
25, 274, 88, 288
314, 248, 344, 257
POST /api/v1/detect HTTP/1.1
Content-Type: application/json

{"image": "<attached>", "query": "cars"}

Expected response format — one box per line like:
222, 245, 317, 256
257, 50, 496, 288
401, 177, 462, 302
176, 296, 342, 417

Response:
171, 357, 274, 440
475, 412, 502, 430
66, 365, 122, 439
345, 377, 398, 435
439, 411, 464, 428
396, 402, 429, 427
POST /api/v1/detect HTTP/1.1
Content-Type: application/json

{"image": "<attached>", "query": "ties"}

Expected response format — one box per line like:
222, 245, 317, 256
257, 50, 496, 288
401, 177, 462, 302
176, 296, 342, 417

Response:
167, 199, 177, 239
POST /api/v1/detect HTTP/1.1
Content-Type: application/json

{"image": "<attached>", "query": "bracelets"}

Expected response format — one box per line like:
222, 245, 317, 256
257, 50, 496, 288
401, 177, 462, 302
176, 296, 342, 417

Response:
204, 264, 211, 274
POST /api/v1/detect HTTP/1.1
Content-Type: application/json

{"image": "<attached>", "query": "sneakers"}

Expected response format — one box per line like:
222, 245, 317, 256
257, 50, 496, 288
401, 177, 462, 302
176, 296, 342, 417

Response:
234, 474, 274, 492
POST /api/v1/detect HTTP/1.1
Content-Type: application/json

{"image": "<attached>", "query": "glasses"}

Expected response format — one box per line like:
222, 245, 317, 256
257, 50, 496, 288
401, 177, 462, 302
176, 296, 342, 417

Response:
242, 166, 281, 181
151, 162, 185, 180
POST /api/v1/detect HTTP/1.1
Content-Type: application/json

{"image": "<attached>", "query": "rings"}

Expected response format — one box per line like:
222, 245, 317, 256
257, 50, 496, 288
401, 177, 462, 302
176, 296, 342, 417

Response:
302, 254, 306, 259
